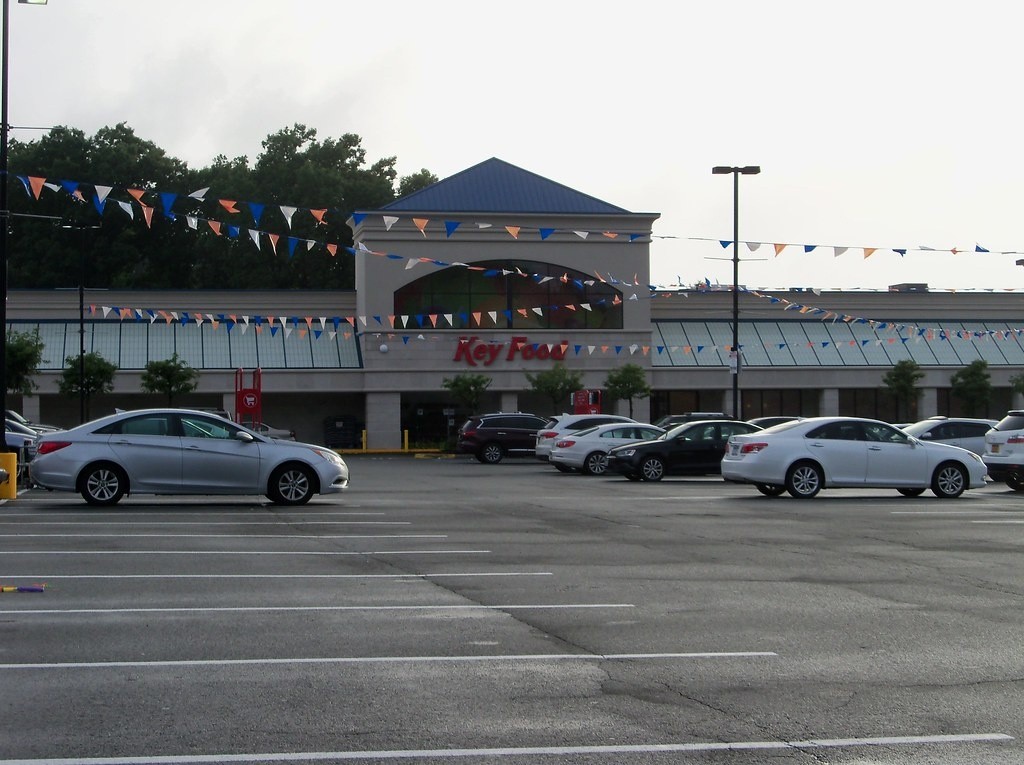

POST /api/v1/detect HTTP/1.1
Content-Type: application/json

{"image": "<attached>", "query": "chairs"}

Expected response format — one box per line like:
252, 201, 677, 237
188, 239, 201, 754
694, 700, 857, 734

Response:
622, 430, 632, 438
826, 426, 842, 439
158, 420, 167, 435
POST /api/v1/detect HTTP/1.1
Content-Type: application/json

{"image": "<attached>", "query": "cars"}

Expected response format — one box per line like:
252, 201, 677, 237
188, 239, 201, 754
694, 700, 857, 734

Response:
5, 410, 61, 484
982, 410, 1024, 492
865, 423, 932, 442
535, 414, 657, 472
237, 421, 298, 442
655, 412, 738, 431
891, 414, 1005, 484
604, 420, 767, 483
747, 416, 797, 431
721, 416, 988, 500
549, 422, 692, 477
28, 408, 350, 509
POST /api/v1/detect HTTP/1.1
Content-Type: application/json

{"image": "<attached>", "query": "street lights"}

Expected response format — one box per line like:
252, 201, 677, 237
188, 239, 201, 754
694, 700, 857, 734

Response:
712, 165, 761, 434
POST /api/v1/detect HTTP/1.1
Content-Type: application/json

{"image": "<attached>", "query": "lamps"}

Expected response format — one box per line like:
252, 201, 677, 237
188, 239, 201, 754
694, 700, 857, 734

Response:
380, 345, 388, 353
631, 344, 640, 353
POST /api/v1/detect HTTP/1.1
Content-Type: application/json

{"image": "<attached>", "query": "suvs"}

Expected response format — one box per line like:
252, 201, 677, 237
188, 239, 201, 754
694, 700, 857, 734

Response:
456, 411, 550, 464
